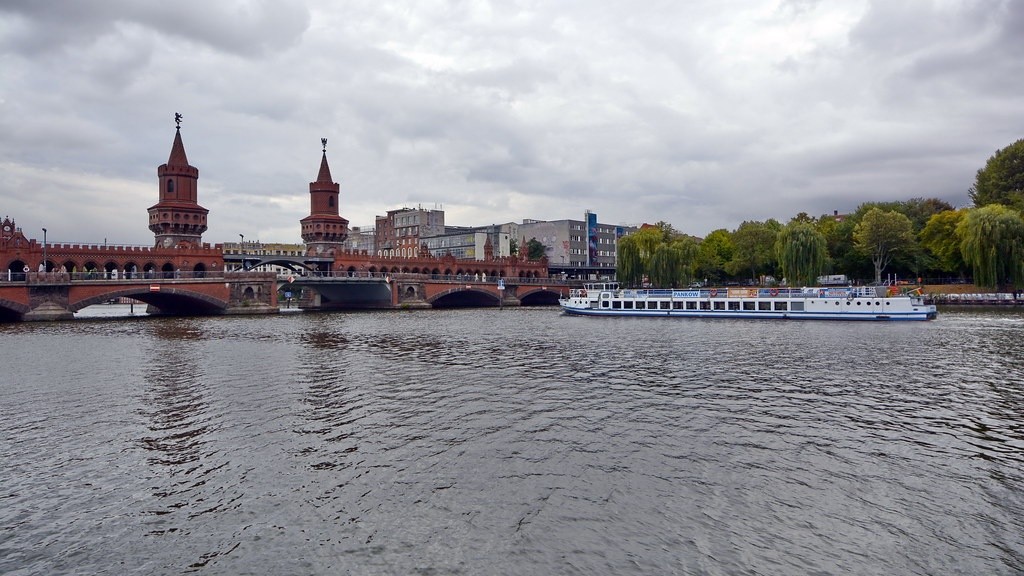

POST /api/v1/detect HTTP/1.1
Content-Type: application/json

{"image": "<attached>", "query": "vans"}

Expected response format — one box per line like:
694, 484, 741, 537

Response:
688, 282, 702, 288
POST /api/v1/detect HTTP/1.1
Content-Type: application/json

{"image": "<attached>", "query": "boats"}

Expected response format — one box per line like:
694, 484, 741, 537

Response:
558, 282, 938, 322
924, 291, 1024, 304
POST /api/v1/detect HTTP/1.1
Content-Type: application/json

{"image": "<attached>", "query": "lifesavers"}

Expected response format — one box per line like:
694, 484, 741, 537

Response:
770, 289, 778, 296
710, 289, 717, 296
931, 299, 935, 305
581, 290, 587, 296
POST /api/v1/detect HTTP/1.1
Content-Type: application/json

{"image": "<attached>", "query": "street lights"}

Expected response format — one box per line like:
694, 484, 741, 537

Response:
41, 228, 47, 272
560, 255, 565, 273
239, 234, 244, 271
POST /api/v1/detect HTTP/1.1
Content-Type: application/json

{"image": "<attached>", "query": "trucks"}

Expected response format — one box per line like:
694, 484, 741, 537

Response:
815, 275, 848, 286
